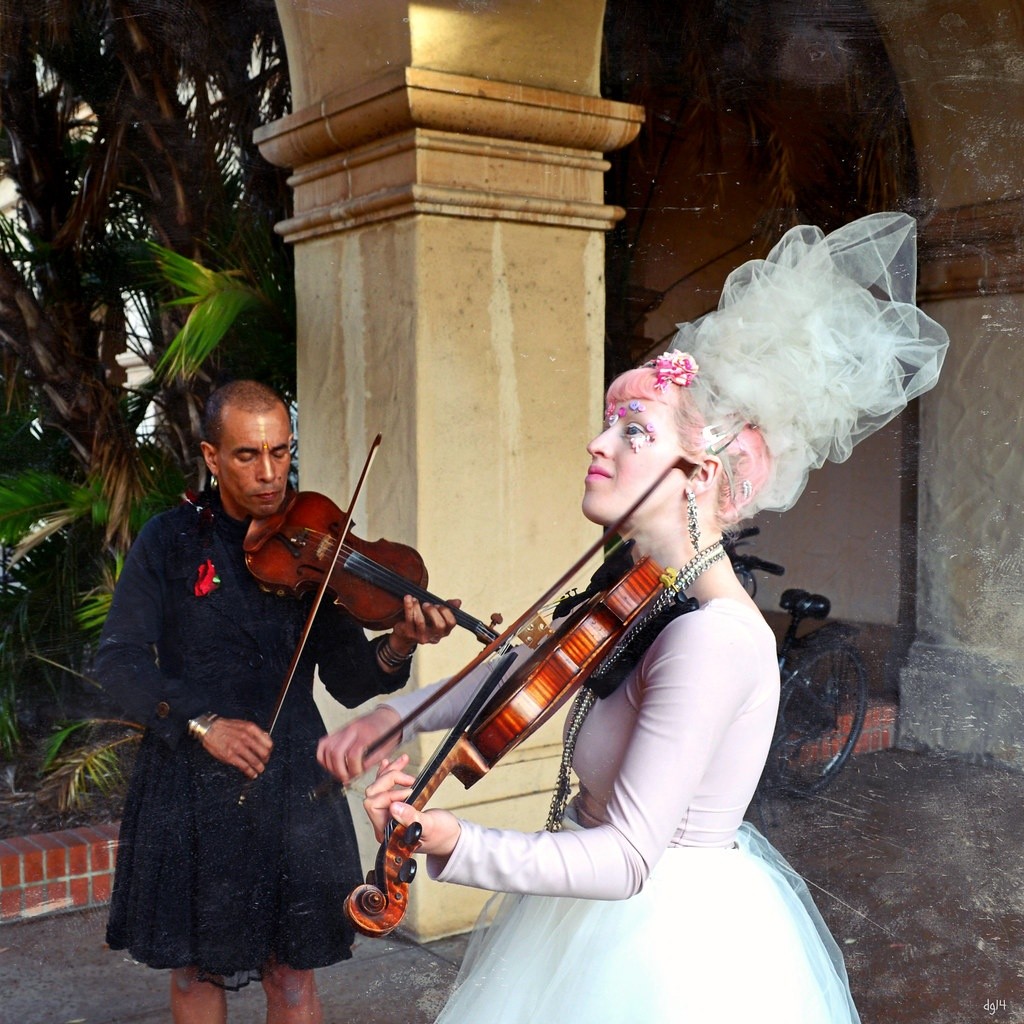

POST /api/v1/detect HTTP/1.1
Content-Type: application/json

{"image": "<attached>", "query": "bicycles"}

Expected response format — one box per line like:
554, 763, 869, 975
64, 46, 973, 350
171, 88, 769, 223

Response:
720, 525, 870, 840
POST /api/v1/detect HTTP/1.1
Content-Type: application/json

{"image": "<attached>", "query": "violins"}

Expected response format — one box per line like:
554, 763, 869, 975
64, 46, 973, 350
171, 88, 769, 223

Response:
240, 487, 511, 654
341, 555, 671, 938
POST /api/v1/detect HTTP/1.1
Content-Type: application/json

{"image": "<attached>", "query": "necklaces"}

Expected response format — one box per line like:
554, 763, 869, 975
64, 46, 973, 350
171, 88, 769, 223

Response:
542, 542, 729, 833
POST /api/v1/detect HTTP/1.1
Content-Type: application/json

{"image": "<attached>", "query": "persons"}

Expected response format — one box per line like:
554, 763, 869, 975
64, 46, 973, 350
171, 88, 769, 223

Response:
94, 378, 462, 1024
316, 213, 951, 1024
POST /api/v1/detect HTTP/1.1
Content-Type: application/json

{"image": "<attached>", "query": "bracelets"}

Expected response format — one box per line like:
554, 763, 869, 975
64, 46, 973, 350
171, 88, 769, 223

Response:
186, 709, 222, 745
